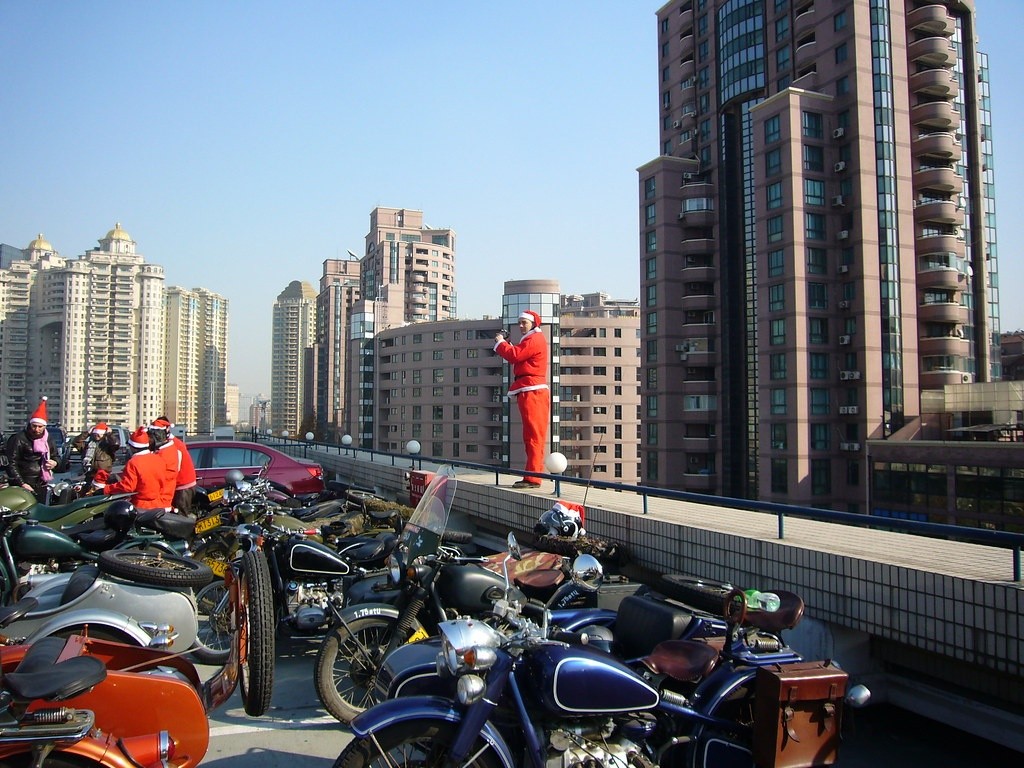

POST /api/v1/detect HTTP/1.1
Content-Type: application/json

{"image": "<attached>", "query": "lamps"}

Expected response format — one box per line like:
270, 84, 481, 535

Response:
306, 432, 314, 449
546, 452, 567, 495
407, 441, 420, 469
341, 434, 352, 455
254, 428, 259, 437
281, 430, 289, 444
266, 428, 272, 441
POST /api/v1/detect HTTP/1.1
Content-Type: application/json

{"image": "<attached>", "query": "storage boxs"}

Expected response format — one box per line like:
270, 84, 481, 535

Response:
579, 575, 644, 612
753, 661, 849, 768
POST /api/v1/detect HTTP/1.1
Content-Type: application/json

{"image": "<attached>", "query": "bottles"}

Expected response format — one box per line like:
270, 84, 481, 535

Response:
733, 588, 780, 612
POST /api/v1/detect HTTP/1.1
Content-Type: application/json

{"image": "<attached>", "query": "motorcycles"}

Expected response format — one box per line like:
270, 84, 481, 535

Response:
268, 462, 871, 768
188, 461, 276, 717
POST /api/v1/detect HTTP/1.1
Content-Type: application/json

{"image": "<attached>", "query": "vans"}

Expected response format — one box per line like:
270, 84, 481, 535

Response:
106, 425, 131, 465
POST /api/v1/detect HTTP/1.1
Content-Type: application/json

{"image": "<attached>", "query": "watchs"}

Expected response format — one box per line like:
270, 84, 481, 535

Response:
53, 461, 57, 466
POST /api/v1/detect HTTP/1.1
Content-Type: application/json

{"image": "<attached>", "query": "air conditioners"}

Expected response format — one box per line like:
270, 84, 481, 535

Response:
574, 465, 579, 468
576, 414, 579, 421
575, 453, 579, 460
675, 344, 699, 464
493, 452, 499, 459
573, 395, 580, 401
683, 173, 692, 179
494, 386, 501, 395
574, 446, 579, 450
692, 110, 698, 135
575, 472, 580, 478
678, 213, 683, 220
493, 432, 499, 440
493, 395, 500, 402
831, 128, 973, 451
663, 102, 669, 109
493, 414, 500, 421
673, 119, 681, 129
576, 433, 579, 440
690, 76, 696, 83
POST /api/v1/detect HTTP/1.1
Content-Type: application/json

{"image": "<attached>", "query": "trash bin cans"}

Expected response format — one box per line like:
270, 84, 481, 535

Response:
214, 428, 235, 441
171, 427, 185, 442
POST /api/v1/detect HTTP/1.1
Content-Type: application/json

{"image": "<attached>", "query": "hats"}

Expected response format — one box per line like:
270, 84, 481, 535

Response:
91, 468, 109, 488
128, 426, 150, 449
149, 419, 176, 431
552, 501, 587, 535
30, 396, 48, 426
519, 310, 542, 332
92, 423, 112, 435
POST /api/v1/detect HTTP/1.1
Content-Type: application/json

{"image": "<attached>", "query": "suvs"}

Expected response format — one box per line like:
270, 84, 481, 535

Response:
46, 424, 71, 473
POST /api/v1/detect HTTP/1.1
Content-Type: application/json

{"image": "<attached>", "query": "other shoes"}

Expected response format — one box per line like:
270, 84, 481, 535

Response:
511, 478, 542, 489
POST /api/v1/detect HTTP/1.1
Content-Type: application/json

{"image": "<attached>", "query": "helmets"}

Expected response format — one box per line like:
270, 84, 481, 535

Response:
105, 499, 138, 534
534, 504, 583, 543
224, 468, 244, 486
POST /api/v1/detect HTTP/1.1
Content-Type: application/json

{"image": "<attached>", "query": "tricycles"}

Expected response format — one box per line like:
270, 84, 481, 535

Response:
0, 623, 209, 768
0, 476, 213, 654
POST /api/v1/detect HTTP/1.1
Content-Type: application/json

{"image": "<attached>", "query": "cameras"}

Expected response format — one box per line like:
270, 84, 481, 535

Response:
501, 331, 508, 340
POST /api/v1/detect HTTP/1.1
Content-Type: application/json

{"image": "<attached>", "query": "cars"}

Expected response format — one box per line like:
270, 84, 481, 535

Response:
65, 431, 83, 455
106, 441, 324, 504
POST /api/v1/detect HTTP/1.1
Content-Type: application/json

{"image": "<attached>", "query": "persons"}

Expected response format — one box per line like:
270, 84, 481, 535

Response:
156, 416, 196, 516
71, 422, 164, 509
151, 420, 178, 508
0, 396, 62, 505
494, 310, 550, 487
